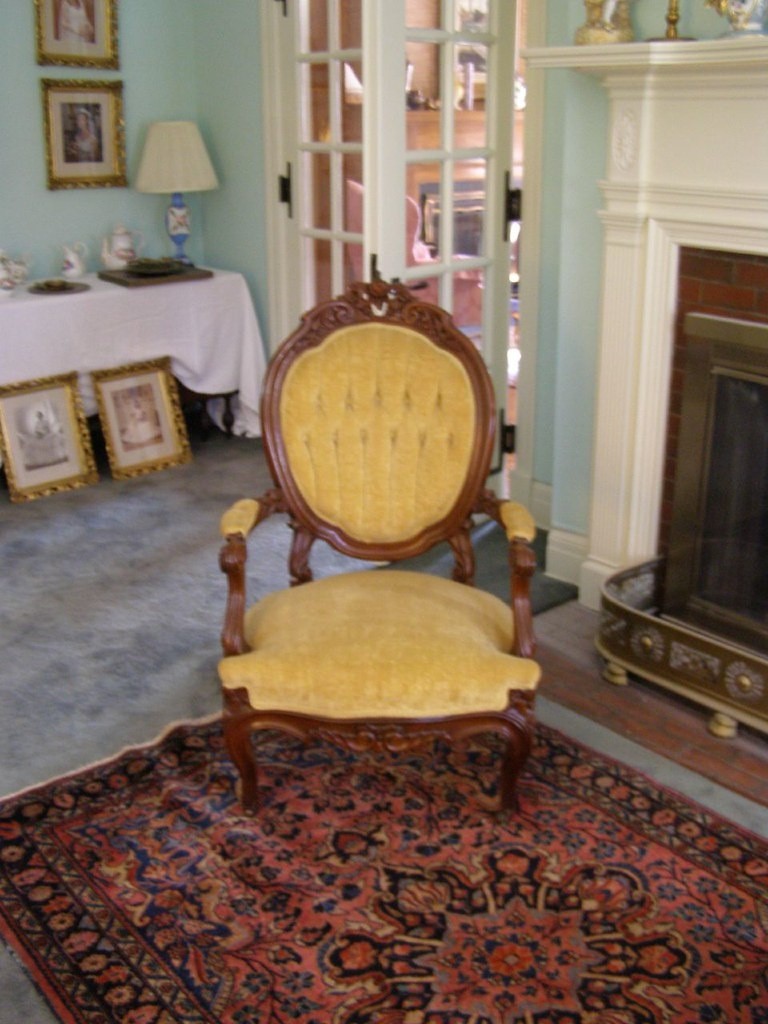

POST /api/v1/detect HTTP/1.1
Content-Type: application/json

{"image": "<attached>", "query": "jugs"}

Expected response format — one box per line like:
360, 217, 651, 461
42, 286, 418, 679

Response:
63, 242, 88, 277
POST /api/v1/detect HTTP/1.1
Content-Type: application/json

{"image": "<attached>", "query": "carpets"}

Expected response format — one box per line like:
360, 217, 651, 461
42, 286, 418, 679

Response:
0, 708, 768, 1024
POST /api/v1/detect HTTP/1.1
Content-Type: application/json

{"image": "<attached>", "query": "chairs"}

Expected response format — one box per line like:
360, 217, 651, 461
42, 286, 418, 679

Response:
345, 179, 484, 326
218, 278, 547, 812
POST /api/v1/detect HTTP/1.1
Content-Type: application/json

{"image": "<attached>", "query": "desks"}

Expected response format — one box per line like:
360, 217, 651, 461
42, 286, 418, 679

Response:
0, 266, 246, 441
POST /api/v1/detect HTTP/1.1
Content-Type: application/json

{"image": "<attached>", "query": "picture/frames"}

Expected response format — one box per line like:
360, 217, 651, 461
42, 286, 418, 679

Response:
34, 0, 119, 70
39, 77, 128, 191
0, 370, 99, 504
452, 0, 488, 99
92, 355, 193, 484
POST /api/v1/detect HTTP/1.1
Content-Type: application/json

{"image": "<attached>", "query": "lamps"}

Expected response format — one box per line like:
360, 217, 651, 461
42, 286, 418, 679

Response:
134, 120, 222, 266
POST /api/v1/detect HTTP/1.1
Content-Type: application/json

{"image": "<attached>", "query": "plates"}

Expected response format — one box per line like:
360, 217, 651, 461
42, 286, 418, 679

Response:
126, 261, 185, 274
28, 283, 89, 294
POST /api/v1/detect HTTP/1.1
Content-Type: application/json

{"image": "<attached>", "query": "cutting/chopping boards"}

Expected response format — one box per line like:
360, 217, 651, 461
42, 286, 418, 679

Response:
99, 267, 213, 285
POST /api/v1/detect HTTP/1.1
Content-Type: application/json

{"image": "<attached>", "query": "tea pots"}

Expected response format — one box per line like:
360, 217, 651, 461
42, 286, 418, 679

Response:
101, 223, 146, 271
